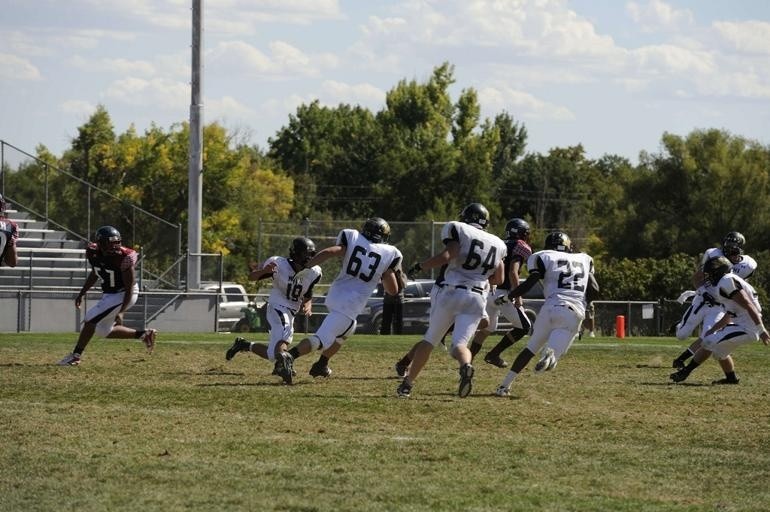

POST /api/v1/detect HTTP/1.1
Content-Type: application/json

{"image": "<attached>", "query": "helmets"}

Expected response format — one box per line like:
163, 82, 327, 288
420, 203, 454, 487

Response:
704, 256, 732, 287
505, 219, 530, 243
544, 231, 571, 254
290, 236, 315, 268
364, 218, 390, 242
459, 203, 489, 229
723, 231, 745, 249
95, 226, 120, 256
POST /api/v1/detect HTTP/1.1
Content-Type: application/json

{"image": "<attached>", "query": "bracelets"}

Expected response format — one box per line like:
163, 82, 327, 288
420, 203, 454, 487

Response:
755, 320, 766, 336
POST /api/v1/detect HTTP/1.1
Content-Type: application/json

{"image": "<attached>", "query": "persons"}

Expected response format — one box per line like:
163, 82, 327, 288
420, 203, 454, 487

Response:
668, 256, 769, 384
272, 216, 403, 382
670, 230, 759, 371
227, 237, 324, 376
0, 198, 19, 268
396, 202, 599, 398
380, 265, 407, 335
58, 224, 158, 365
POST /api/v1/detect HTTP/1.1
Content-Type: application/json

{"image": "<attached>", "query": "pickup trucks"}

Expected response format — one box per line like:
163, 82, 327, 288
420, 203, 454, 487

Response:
178, 279, 269, 333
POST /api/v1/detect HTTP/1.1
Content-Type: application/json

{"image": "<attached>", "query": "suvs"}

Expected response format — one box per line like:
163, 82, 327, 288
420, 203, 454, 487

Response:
476, 278, 548, 338
353, 277, 439, 335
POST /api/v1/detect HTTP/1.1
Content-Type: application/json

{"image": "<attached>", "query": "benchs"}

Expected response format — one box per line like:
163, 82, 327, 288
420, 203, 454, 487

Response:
0, 197, 160, 289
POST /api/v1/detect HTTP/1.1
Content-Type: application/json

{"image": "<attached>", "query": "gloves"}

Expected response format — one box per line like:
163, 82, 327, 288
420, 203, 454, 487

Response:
407, 264, 420, 282
494, 296, 508, 306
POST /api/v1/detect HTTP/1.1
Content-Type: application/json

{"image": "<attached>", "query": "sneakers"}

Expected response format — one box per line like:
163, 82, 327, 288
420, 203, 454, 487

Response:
458, 365, 474, 397
485, 352, 507, 367
226, 336, 244, 360
273, 351, 296, 384
533, 349, 555, 373
670, 318, 740, 384
139, 328, 157, 356
396, 360, 413, 397
57, 353, 81, 366
309, 364, 331, 379
496, 386, 510, 397
